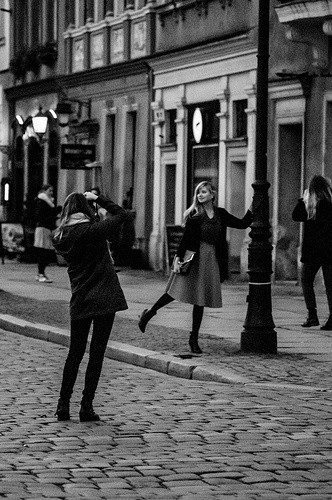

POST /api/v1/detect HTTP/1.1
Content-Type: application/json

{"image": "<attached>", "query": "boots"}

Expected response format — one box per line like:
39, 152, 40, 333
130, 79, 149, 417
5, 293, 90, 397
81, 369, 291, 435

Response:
189, 332, 203, 353
320, 314, 332, 331
138, 309, 157, 333
301, 309, 320, 327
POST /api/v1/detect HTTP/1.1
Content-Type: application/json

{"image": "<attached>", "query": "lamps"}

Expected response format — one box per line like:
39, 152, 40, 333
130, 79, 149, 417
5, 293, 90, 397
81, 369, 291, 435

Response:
33, 105, 69, 144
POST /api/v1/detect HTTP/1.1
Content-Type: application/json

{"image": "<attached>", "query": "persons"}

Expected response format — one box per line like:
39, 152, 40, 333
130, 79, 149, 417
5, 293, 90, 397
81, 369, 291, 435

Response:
50, 191, 129, 423
28, 186, 63, 283
139, 181, 253, 354
83, 187, 103, 223
292, 176, 332, 330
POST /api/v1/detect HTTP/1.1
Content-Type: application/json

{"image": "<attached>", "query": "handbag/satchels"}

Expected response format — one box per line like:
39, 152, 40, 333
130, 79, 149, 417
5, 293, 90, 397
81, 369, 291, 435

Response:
171, 248, 197, 276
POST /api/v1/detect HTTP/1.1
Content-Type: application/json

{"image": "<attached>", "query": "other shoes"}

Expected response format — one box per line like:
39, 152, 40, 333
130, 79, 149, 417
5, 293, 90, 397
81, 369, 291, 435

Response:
54, 403, 71, 422
79, 407, 100, 422
35, 273, 53, 284
